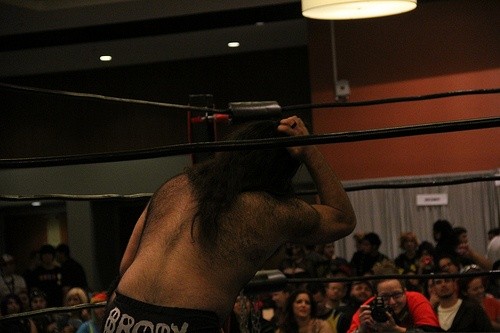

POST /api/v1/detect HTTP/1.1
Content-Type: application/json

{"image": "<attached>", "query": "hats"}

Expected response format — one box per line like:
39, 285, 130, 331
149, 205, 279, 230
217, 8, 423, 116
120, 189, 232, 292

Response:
486, 234, 500, 265
90, 291, 108, 303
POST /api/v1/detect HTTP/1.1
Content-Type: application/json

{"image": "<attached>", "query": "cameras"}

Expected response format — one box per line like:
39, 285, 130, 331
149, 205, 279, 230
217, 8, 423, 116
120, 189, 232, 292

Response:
366, 297, 392, 323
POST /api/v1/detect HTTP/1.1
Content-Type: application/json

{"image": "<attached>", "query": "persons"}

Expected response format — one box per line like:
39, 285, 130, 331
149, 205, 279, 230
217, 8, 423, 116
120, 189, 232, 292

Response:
0, 218, 500, 333
99, 116, 357, 333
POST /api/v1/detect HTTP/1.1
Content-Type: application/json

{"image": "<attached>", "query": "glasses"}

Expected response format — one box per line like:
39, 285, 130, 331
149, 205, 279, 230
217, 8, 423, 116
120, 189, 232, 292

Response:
464, 263, 480, 273
378, 290, 403, 300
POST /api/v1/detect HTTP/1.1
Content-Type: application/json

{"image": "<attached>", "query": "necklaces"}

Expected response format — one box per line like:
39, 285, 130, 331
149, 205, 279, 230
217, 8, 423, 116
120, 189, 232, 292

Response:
439, 311, 455, 329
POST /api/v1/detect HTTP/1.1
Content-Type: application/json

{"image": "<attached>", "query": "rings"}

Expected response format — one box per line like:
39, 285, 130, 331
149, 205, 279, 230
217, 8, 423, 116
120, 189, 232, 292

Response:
290, 121, 297, 129
